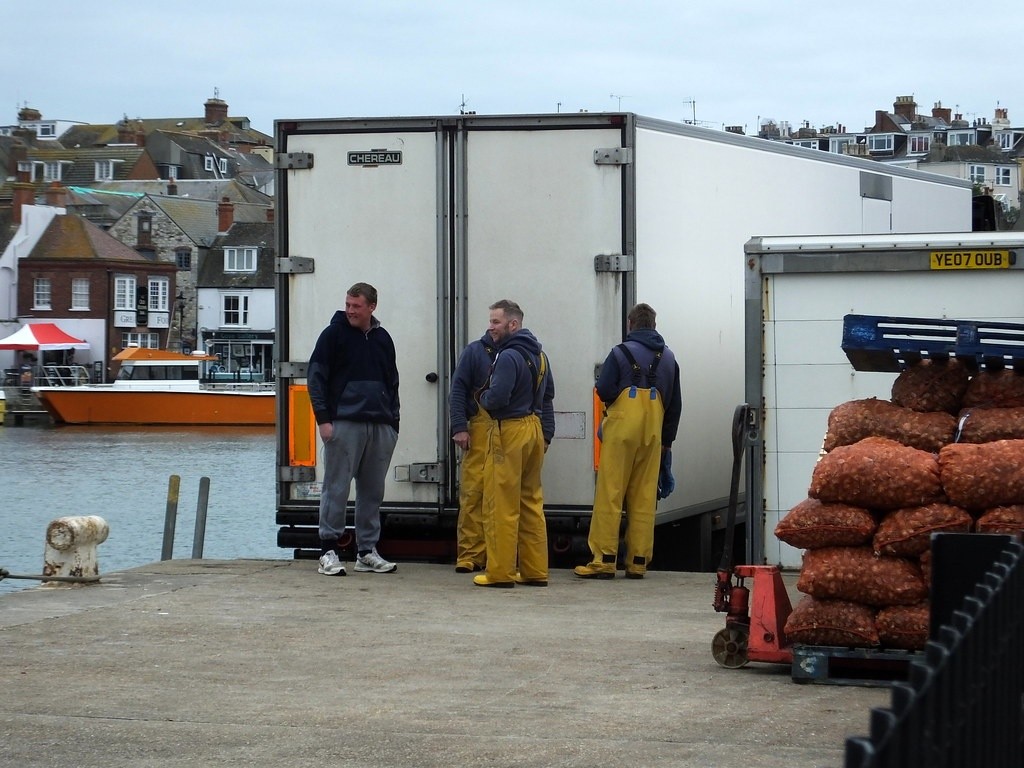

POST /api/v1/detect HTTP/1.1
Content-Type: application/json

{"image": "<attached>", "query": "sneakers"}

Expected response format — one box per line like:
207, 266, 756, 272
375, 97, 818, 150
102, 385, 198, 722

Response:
317, 550, 347, 576
353, 547, 398, 572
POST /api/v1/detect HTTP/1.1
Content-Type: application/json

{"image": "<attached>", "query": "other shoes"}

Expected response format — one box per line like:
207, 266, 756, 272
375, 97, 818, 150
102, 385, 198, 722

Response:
473, 574, 515, 588
516, 572, 548, 587
625, 572, 643, 579
455, 562, 482, 573
574, 566, 615, 580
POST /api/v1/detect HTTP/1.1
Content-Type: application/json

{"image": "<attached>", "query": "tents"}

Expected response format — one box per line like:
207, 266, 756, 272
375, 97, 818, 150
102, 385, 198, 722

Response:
1, 323, 90, 388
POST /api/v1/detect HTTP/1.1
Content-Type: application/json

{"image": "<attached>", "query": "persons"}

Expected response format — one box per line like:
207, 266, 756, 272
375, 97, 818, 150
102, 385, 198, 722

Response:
308, 283, 401, 576
574, 303, 683, 579
448, 303, 554, 574
473, 299, 548, 589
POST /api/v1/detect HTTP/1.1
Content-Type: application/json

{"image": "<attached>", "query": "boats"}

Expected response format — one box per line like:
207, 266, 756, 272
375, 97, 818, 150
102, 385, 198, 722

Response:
29, 345, 277, 425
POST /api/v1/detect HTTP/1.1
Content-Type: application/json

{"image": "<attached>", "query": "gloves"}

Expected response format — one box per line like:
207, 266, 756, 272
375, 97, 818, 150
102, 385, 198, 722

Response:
656, 446, 675, 500
